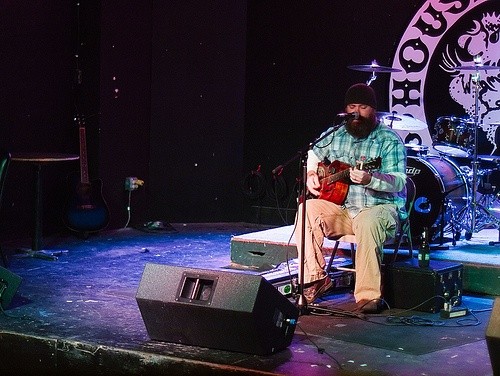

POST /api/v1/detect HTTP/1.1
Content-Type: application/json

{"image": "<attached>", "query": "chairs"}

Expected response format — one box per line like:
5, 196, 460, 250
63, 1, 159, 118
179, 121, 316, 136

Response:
326, 177, 416, 313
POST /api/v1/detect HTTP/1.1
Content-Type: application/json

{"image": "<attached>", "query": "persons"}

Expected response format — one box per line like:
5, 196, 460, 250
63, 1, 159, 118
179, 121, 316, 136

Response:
294, 84, 408, 310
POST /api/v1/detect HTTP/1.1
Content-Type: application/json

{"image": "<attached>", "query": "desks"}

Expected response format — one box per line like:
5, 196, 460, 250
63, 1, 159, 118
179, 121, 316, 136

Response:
8, 144, 80, 260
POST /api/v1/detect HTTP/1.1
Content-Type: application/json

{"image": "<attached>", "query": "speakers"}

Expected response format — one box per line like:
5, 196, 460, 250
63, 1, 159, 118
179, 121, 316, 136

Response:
0, 263, 21, 310
485, 297, 500, 376
136, 263, 299, 355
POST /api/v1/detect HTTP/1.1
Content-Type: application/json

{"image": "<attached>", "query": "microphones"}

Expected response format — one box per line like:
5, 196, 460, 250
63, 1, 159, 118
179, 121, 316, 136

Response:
337, 111, 359, 119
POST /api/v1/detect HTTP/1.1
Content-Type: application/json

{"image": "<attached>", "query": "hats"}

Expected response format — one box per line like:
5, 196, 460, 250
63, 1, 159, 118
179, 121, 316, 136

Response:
344, 83, 377, 112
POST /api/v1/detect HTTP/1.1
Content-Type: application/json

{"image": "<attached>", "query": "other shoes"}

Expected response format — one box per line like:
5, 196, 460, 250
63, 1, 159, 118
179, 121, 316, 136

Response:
358, 299, 379, 315
299, 275, 332, 307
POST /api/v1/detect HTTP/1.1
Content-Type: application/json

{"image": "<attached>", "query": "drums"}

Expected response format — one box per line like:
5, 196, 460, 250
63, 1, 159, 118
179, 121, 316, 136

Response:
431, 115, 475, 159
404, 154, 469, 240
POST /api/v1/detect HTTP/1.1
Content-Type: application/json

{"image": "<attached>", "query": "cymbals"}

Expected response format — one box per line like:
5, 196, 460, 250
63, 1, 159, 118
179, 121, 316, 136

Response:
449, 65, 500, 70
375, 113, 428, 131
347, 64, 403, 72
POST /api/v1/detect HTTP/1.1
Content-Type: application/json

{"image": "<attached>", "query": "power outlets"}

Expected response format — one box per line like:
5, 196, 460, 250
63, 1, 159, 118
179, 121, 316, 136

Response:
125, 177, 139, 191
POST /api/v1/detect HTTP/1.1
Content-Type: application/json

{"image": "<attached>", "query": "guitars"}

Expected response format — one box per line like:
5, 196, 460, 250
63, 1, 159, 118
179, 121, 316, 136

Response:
64, 113, 111, 232
295, 155, 382, 204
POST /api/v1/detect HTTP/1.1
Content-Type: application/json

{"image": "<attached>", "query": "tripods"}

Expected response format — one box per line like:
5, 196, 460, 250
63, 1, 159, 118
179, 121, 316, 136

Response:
431, 72, 500, 246
272, 122, 365, 320
